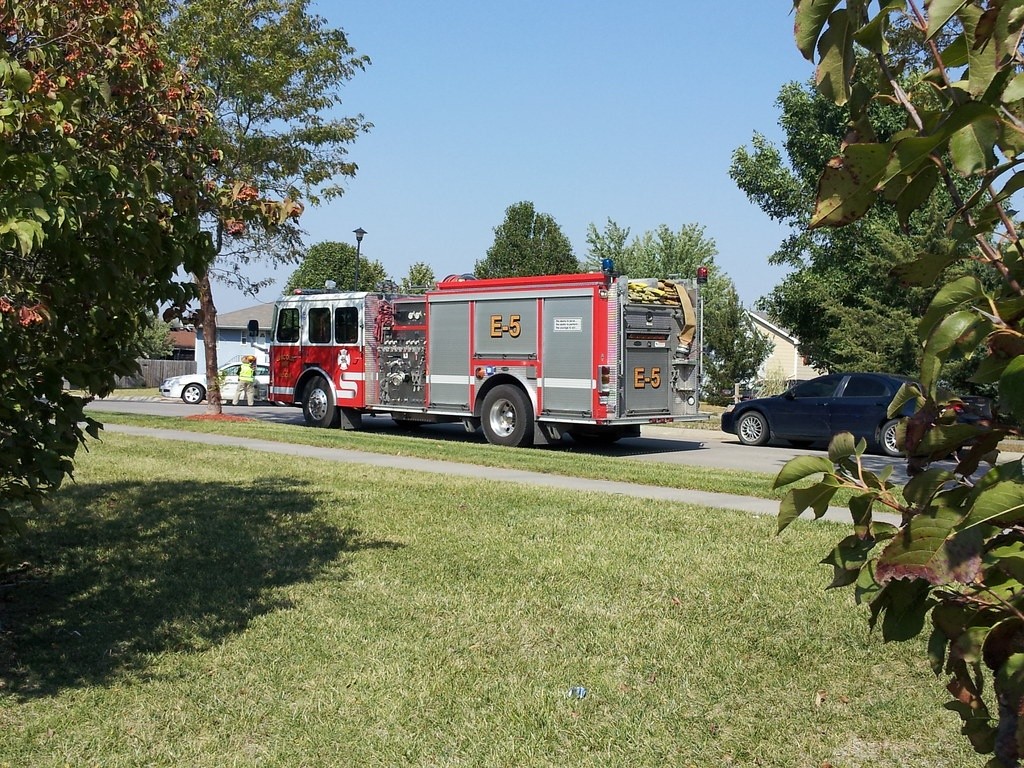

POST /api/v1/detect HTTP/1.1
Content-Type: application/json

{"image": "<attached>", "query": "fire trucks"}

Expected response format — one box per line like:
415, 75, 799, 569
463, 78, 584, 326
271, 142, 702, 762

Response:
268, 259, 712, 448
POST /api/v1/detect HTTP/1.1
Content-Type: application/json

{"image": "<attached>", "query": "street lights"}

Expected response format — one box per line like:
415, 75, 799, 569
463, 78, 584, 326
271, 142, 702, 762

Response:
352, 227, 368, 292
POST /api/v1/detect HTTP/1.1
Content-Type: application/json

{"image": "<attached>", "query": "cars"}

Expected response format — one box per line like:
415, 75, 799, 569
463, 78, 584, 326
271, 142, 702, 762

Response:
721, 379, 807, 403
160, 363, 270, 405
720, 372, 991, 457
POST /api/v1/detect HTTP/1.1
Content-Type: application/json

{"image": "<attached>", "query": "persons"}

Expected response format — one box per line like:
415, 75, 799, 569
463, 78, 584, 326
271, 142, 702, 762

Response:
228, 357, 255, 407
